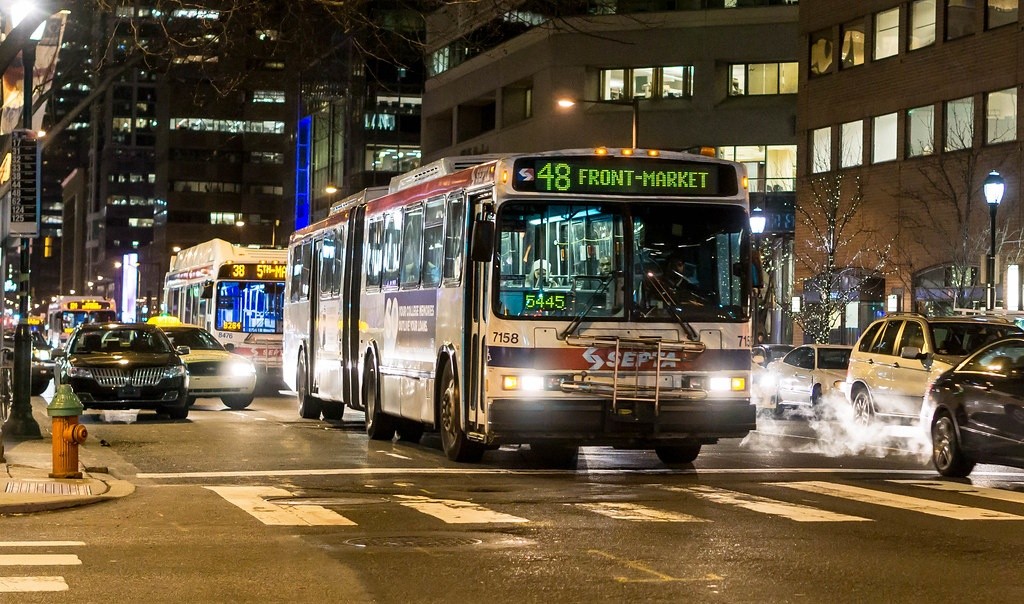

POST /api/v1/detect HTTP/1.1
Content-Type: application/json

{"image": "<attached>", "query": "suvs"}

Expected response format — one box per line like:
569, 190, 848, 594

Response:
1, 330, 58, 395
844, 311, 1024, 432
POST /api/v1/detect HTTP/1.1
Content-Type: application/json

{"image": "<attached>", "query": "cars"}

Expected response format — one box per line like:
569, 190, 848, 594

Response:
51, 322, 192, 421
148, 314, 256, 410
751, 342, 796, 383
921, 337, 1024, 476
766, 343, 855, 417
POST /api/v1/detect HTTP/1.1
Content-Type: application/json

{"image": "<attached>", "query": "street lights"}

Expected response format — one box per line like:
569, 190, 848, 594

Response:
983, 170, 1004, 310
558, 97, 638, 148
135, 262, 160, 315
5, 0, 49, 440
325, 184, 371, 194
115, 262, 142, 298
236, 220, 275, 246
749, 206, 767, 345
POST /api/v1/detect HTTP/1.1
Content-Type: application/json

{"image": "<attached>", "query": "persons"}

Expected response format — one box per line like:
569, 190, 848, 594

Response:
980, 332, 1000, 347
938, 340, 959, 355
581, 255, 613, 291
524, 259, 558, 287
647, 252, 705, 305
754, 331, 766, 345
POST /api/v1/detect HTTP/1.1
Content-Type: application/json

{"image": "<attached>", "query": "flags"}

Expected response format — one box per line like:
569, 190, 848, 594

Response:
0, 10, 68, 135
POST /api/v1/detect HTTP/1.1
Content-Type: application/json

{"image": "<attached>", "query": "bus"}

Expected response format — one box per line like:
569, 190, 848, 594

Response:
31, 296, 116, 354
282, 147, 764, 467
163, 238, 288, 393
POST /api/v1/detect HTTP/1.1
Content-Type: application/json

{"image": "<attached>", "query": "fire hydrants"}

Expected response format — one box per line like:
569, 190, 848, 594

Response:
47, 384, 87, 479
